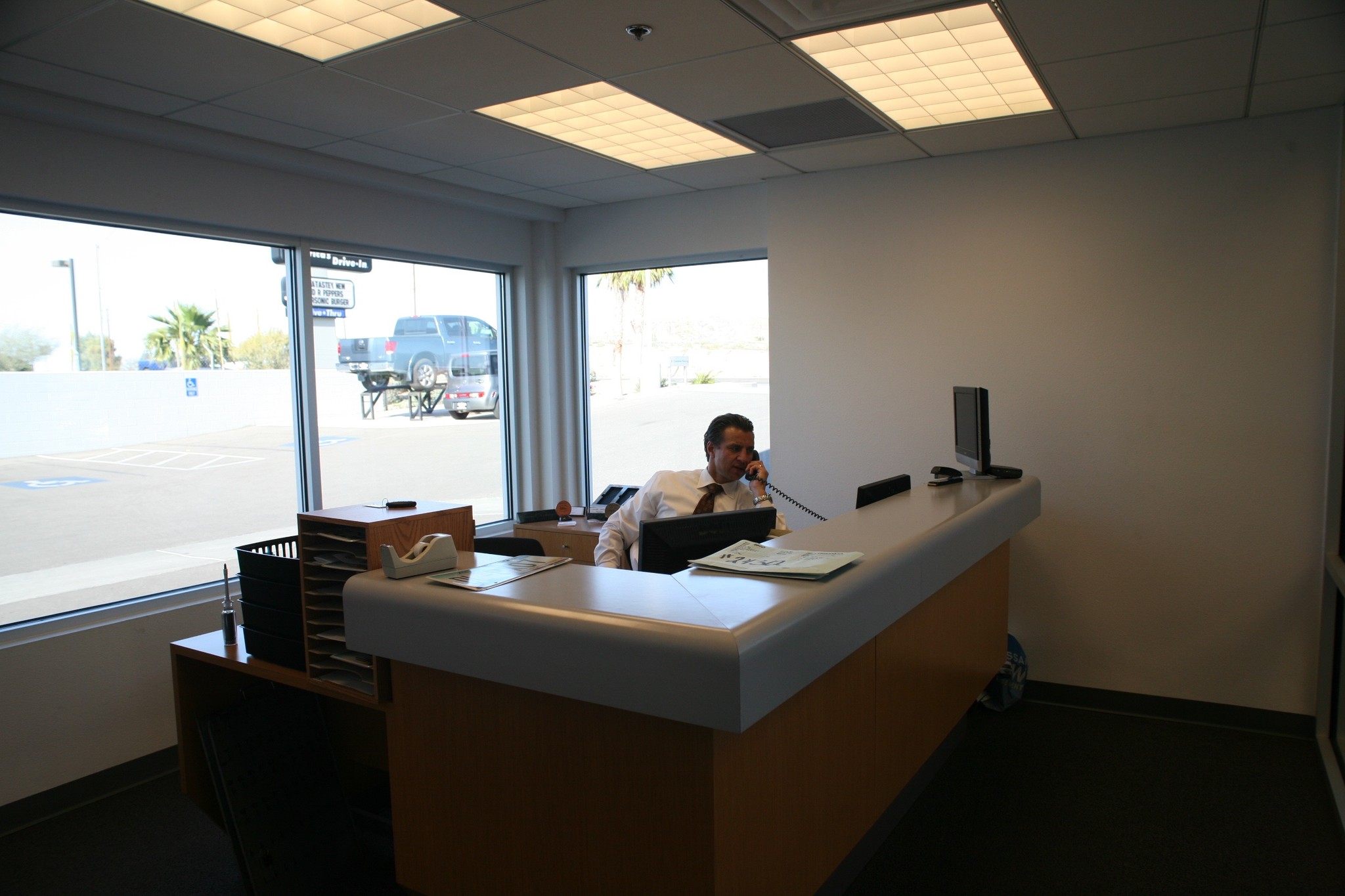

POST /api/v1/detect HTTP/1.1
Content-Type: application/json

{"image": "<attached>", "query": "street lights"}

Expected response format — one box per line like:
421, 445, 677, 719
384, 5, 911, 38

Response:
50, 258, 83, 372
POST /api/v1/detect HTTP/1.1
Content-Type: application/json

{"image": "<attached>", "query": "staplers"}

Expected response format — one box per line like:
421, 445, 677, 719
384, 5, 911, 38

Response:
927, 466, 963, 486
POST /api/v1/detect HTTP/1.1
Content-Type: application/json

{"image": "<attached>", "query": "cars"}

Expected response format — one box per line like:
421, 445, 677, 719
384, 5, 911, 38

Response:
137, 346, 209, 371
443, 350, 500, 419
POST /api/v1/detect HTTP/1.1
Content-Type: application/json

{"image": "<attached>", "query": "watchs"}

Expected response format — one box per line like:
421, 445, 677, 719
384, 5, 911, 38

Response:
753, 493, 773, 505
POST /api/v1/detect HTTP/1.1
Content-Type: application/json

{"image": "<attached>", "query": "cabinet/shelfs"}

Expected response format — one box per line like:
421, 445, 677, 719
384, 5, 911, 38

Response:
296, 506, 475, 702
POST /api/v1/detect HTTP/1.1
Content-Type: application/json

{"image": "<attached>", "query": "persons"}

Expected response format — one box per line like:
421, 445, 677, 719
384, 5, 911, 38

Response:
593, 413, 794, 569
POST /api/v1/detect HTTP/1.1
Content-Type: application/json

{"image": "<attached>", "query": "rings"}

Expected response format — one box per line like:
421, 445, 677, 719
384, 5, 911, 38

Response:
759, 465, 763, 467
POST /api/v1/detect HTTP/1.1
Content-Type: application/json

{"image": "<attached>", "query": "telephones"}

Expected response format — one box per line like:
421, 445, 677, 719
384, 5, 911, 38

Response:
745, 450, 760, 481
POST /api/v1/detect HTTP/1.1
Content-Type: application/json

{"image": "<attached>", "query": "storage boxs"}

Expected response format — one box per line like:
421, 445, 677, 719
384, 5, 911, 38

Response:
239, 535, 305, 670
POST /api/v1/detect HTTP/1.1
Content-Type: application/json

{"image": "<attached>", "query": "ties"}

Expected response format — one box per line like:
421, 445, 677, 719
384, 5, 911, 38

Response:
692, 484, 724, 514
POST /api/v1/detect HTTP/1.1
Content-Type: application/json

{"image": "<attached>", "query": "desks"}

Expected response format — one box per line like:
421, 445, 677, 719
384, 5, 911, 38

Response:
343, 471, 1041, 896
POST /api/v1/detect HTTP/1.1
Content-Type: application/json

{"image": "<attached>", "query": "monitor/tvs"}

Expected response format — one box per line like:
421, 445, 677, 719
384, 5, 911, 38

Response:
855, 474, 911, 509
952, 386, 1023, 479
638, 507, 777, 575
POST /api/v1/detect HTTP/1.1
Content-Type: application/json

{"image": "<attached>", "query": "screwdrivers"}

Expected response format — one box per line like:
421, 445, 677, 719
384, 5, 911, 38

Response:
221, 563, 238, 646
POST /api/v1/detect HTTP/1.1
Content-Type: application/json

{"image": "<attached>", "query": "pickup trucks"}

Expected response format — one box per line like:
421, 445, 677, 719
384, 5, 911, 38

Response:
336, 314, 497, 394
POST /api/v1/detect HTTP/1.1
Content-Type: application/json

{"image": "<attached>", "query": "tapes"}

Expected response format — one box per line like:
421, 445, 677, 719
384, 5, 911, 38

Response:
414, 542, 429, 557
